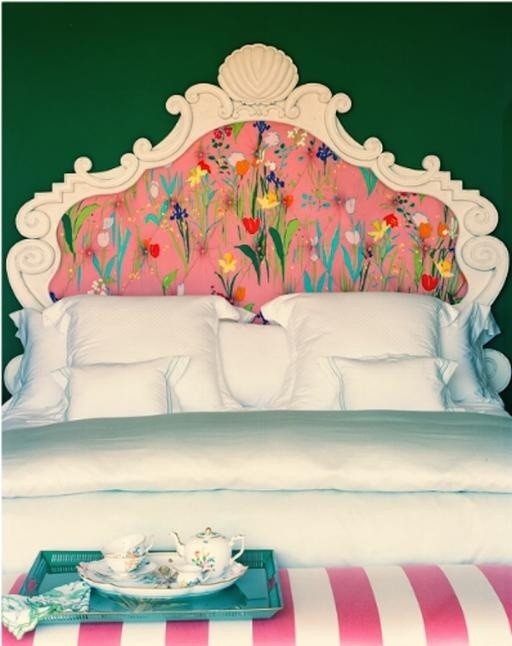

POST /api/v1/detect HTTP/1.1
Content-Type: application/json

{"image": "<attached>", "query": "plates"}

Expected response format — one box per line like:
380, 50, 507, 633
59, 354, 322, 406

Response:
78, 553, 248, 600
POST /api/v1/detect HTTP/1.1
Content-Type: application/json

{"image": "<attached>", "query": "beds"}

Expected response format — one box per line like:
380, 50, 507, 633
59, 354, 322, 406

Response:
0, 43, 512, 563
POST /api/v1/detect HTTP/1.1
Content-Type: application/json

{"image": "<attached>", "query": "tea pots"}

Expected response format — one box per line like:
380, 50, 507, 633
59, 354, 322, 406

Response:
167, 525, 245, 581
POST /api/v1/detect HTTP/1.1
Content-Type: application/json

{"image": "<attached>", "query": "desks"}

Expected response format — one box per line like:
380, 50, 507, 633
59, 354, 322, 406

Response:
0, 561, 512, 646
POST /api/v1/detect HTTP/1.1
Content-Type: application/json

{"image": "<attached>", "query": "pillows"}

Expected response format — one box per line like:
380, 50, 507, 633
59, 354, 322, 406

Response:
321, 352, 460, 412
54, 360, 190, 417
42, 293, 244, 408
1, 305, 505, 416
262, 289, 466, 413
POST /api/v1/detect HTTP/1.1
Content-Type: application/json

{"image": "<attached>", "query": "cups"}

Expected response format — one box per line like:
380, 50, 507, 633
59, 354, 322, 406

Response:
101, 534, 156, 575
